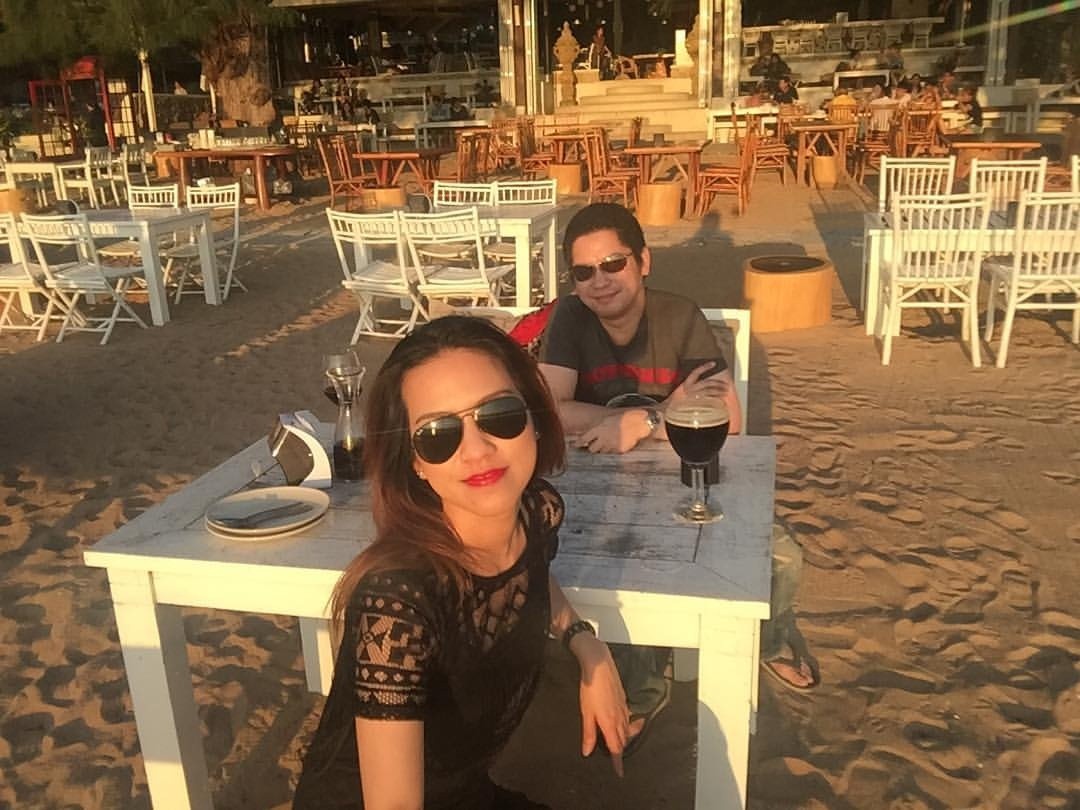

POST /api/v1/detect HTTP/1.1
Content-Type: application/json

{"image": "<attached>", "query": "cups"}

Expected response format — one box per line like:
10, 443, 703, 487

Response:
681, 453, 719, 487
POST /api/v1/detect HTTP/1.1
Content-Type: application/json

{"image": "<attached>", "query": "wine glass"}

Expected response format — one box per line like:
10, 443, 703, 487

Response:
321, 352, 361, 463
665, 395, 730, 523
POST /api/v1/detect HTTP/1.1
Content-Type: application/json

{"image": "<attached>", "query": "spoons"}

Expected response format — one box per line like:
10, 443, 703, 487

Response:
214, 503, 312, 528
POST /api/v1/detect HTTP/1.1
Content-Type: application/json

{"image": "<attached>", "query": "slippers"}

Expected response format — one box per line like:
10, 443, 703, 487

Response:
759, 637, 821, 694
597, 679, 672, 759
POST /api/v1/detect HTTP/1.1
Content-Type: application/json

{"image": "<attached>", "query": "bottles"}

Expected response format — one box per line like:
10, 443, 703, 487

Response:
326, 367, 367, 481
318, 121, 322, 132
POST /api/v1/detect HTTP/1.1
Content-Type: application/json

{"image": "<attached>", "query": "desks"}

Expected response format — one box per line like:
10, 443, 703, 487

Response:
941, 133, 1042, 196
708, 105, 779, 142
381, 85, 432, 111
861, 209, 1080, 337
354, 147, 447, 192
290, 128, 371, 177
294, 97, 337, 116
542, 130, 614, 163
84, 420, 778, 810
633, 52, 674, 78
625, 137, 713, 216
6, 158, 82, 208
350, 203, 562, 329
832, 69, 890, 91
908, 108, 960, 116
157, 148, 298, 210
413, 121, 487, 148
10, 209, 222, 328
789, 121, 859, 186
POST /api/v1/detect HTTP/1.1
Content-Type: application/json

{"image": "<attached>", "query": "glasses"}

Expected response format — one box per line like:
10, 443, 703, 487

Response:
411, 396, 531, 464
570, 247, 638, 283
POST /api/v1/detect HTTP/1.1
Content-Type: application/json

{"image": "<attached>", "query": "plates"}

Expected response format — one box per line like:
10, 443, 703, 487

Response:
204, 486, 330, 535
206, 513, 328, 542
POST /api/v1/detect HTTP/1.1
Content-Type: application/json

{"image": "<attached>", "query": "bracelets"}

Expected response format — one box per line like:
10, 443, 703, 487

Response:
561, 620, 595, 655
647, 409, 659, 431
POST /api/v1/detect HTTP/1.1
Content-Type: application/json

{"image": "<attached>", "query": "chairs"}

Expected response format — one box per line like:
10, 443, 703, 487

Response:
0, 103, 1080, 677
742, 21, 934, 56
576, 42, 595, 70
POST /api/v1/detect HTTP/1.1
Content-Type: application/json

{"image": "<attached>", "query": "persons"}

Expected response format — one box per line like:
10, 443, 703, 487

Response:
426, 94, 447, 148
768, 53, 792, 80
423, 85, 433, 111
361, 98, 380, 124
750, 56, 767, 75
80, 97, 109, 174
773, 76, 799, 103
447, 96, 470, 147
919, 66, 983, 135
472, 79, 494, 103
835, 41, 904, 88
338, 100, 359, 124
291, 315, 631, 810
755, 73, 775, 94
534, 201, 822, 759
311, 74, 353, 113
820, 73, 927, 151
